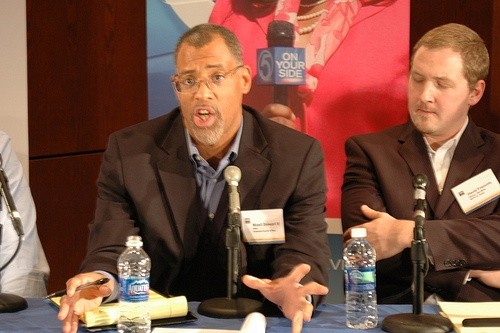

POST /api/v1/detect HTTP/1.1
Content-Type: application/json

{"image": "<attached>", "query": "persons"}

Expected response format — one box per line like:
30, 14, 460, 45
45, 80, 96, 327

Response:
207, 0, 411, 218
0, 129, 50, 297
57, 23, 329, 333
340, 23, 500, 304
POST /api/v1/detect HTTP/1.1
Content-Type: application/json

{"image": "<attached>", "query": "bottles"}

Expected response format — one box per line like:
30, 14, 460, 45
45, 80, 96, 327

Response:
343, 228, 378, 329
117, 236, 151, 333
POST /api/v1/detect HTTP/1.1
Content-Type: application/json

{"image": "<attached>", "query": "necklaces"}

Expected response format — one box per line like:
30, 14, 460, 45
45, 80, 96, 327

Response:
297, 0, 326, 34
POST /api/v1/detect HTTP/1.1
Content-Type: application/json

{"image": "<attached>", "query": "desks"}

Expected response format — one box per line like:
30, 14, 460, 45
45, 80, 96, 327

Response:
0, 297, 455, 333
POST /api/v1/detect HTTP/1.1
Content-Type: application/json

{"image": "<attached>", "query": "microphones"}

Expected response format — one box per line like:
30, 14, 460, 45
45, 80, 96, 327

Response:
257, 21, 306, 107
0, 154, 25, 237
223, 165, 242, 243
410, 174, 428, 232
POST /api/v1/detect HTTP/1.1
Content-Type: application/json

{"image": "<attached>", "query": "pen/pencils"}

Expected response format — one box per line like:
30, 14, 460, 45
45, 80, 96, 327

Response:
44, 278, 110, 299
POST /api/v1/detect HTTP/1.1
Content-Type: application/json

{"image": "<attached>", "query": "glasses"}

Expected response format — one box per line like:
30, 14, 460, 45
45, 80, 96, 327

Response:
173, 66, 236, 93
